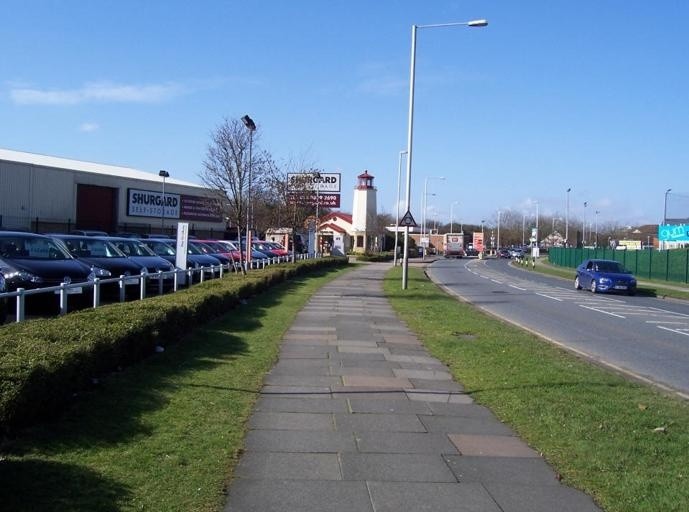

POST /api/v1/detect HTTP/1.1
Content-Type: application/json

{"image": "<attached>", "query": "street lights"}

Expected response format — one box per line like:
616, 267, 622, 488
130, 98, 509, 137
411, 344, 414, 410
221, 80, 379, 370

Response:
242, 114, 257, 272
399, 18, 489, 290
390, 148, 410, 266
662, 187, 675, 250
419, 174, 446, 258
450, 198, 561, 252
561, 184, 601, 249
313, 170, 323, 261
158, 169, 170, 230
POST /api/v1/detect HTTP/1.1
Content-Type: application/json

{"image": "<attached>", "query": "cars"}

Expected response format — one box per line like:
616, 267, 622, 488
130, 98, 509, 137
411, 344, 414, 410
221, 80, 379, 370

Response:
573, 256, 641, 298
497, 245, 525, 260
0, 230, 290, 317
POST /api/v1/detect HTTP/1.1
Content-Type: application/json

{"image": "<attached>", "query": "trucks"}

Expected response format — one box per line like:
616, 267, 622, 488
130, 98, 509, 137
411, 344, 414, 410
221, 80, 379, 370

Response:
440, 230, 464, 259
